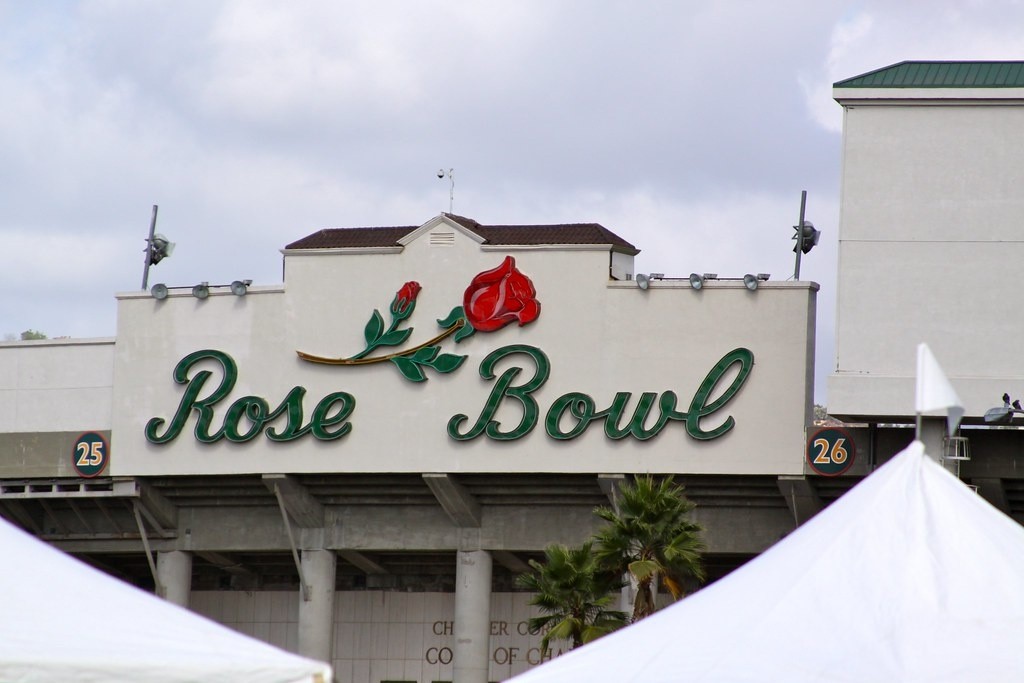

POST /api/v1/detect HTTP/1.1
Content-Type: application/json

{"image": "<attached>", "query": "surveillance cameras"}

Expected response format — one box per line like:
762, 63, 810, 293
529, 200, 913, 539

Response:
437, 171, 445, 178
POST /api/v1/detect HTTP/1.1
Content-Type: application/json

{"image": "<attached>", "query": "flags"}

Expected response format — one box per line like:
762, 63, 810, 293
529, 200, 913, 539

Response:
918, 340, 965, 439
